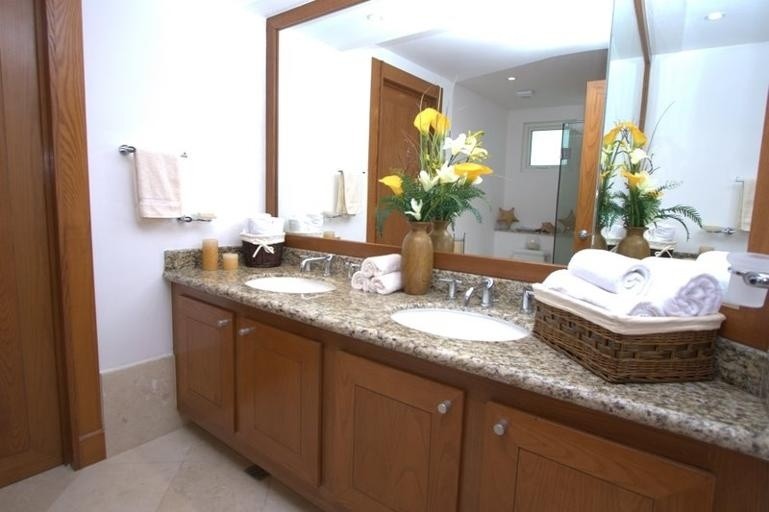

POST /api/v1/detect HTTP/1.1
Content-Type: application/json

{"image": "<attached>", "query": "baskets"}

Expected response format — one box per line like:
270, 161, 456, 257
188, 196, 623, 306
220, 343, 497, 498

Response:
240, 232, 285, 268
531, 282, 727, 383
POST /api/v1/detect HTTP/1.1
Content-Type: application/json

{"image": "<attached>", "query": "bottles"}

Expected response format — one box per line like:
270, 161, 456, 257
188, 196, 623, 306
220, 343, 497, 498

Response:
202, 237, 218, 272
222, 253, 238, 270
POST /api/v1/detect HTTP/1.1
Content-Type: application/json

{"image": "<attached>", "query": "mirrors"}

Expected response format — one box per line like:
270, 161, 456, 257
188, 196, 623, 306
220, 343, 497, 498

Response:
267, 0, 769, 350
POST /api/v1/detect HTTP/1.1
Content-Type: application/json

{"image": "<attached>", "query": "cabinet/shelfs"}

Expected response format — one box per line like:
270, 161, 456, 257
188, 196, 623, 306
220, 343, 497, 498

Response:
343, 339, 769, 511
170, 282, 323, 512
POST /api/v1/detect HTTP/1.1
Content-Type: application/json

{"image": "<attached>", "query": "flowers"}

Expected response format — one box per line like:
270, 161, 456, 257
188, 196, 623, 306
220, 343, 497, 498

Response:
375, 80, 490, 240
592, 128, 626, 224
615, 120, 702, 244
434, 130, 493, 220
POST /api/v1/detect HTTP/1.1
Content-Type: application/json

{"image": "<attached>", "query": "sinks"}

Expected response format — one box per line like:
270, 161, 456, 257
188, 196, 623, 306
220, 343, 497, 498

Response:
244, 276, 337, 294
392, 308, 531, 341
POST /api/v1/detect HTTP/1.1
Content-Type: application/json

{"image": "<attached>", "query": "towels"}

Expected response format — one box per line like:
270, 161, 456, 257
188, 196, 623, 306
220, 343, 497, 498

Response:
741, 177, 755, 234
369, 274, 403, 293
336, 170, 361, 218
362, 253, 401, 275
134, 146, 182, 218
545, 248, 722, 317
351, 271, 370, 292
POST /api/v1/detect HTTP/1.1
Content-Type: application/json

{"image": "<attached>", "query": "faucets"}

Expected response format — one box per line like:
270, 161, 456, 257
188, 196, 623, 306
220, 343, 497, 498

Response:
301, 254, 336, 277
464, 278, 495, 307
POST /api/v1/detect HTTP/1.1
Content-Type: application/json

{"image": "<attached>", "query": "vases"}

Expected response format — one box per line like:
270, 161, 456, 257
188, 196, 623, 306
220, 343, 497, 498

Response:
429, 218, 454, 253
590, 222, 608, 250
617, 227, 652, 258
401, 221, 436, 295
